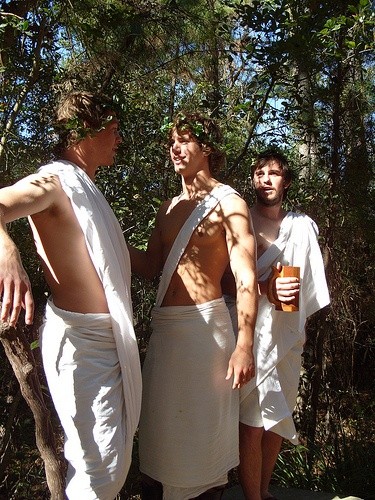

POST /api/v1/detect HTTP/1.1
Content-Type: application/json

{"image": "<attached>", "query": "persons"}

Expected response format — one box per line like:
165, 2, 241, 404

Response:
0, 92, 144, 500
125, 109, 259, 500
220, 150, 331, 500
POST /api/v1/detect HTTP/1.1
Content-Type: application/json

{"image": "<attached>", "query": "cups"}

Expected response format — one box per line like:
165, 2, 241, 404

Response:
269, 265, 300, 312
